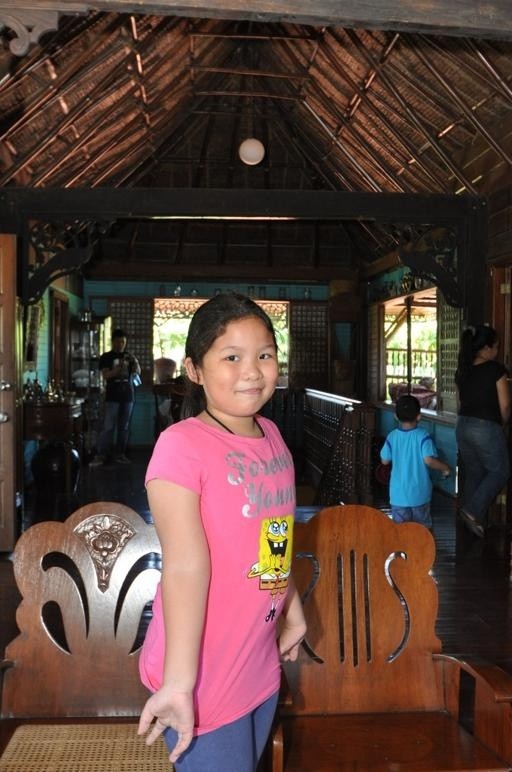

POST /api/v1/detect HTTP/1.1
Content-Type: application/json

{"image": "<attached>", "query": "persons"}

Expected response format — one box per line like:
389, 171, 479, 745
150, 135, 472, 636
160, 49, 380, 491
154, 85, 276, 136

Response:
88, 328, 141, 467
454, 325, 512, 541
378, 395, 451, 531
136, 291, 312, 772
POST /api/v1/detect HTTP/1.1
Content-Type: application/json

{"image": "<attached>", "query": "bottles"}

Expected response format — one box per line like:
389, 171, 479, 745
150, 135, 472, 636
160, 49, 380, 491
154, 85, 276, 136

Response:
132, 371, 142, 387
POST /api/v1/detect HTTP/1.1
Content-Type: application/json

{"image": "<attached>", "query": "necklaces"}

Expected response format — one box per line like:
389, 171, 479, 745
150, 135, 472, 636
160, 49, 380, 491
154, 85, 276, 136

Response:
204, 407, 266, 438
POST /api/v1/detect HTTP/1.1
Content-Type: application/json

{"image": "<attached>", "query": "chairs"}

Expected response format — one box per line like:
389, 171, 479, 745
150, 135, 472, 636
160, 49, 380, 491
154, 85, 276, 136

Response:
2, 509, 177, 772
271, 507, 509, 771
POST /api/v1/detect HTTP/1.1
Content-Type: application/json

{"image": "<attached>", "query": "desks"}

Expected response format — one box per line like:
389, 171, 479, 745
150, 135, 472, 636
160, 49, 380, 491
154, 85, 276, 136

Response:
24, 397, 87, 516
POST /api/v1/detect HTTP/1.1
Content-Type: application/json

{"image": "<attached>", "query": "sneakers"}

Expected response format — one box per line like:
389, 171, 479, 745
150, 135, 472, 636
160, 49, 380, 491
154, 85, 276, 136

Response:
456, 504, 485, 539
115, 453, 130, 465
88, 454, 103, 466
